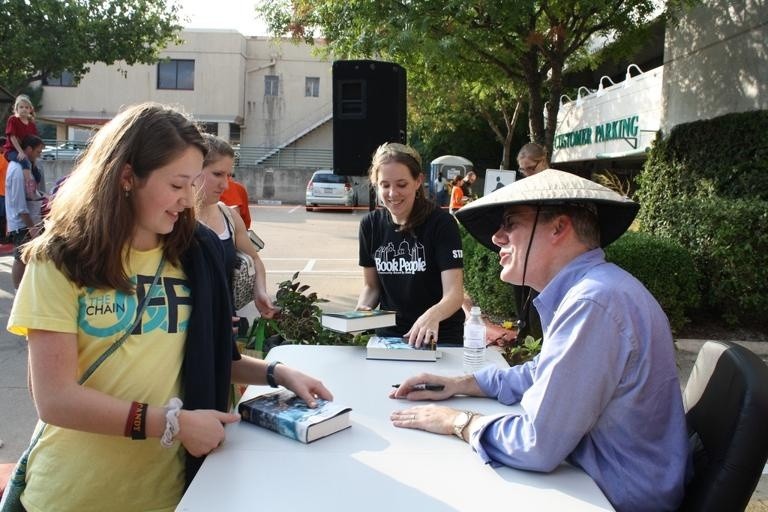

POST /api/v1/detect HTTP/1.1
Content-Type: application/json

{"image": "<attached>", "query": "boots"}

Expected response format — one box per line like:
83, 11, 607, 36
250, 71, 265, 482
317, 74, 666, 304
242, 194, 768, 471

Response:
25, 179, 45, 201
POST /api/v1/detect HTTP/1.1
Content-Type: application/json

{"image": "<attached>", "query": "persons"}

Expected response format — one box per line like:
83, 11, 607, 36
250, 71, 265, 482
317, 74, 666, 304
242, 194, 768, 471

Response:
190, 132, 275, 335
389, 165, 691, 512
433, 171, 476, 215
0, 94, 46, 245
0, 102, 333, 512
510, 137, 549, 355
352, 142, 464, 348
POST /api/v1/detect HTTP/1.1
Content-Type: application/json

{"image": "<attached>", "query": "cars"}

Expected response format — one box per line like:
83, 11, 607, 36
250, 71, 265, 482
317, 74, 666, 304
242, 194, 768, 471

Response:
306, 170, 360, 211
40, 143, 88, 161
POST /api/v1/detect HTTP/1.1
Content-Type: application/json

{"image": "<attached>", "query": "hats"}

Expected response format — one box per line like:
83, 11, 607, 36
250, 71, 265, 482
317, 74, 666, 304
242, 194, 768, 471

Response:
454, 169, 641, 254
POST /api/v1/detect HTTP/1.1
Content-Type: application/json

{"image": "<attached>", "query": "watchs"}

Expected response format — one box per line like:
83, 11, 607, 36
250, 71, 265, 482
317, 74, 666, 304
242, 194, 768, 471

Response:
452, 409, 483, 440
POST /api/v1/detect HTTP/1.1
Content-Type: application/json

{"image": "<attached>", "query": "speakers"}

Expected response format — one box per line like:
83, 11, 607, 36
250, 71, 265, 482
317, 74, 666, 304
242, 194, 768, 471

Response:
332, 60, 406, 177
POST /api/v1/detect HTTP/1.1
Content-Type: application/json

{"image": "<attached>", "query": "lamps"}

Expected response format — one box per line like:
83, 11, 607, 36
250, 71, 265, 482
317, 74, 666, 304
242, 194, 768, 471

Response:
542, 63, 647, 119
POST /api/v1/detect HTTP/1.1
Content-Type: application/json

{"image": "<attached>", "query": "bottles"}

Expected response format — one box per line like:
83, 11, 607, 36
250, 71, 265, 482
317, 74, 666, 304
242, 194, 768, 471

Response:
460, 305, 489, 372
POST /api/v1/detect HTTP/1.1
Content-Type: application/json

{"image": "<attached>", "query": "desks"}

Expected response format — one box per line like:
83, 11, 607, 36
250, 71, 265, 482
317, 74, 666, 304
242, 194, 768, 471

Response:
168, 345, 616, 512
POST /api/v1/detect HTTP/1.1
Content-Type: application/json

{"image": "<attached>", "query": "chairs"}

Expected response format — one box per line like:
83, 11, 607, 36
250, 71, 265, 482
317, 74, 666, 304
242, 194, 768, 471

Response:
682, 338, 768, 512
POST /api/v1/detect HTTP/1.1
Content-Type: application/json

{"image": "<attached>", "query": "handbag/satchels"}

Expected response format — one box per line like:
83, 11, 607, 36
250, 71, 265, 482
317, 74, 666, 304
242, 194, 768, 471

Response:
232, 248, 256, 310
0, 451, 28, 512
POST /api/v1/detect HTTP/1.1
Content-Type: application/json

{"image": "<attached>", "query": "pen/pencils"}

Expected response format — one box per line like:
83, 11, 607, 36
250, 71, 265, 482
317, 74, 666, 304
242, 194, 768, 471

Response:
392, 383, 446, 390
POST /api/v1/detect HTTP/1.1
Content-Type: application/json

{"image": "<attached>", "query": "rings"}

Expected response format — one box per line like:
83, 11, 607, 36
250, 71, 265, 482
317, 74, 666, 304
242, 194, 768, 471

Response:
410, 414, 417, 420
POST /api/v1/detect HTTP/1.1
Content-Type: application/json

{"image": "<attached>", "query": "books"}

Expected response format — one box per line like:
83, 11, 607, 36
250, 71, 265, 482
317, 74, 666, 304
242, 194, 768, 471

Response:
237, 387, 354, 445
365, 335, 439, 362
319, 308, 399, 334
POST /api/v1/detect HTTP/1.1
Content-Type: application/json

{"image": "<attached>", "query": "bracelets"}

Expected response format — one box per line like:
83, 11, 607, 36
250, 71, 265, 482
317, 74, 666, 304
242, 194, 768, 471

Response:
129, 402, 148, 440
123, 400, 139, 437
159, 397, 184, 449
266, 360, 284, 388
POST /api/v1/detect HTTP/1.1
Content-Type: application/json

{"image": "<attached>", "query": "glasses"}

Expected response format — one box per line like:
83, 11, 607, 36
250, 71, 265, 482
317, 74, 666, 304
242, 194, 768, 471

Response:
519, 162, 539, 171
